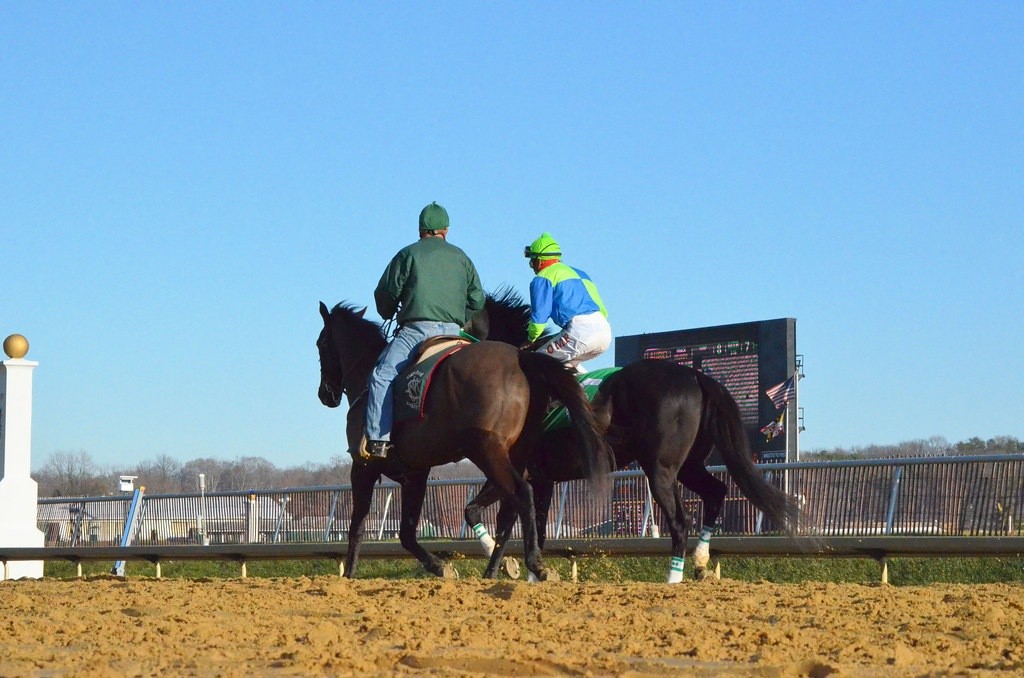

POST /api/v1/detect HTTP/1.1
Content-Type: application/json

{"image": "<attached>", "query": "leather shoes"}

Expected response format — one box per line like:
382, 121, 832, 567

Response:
349, 439, 389, 458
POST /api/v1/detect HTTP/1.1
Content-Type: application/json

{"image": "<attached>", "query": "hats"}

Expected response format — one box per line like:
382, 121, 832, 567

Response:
529, 232, 562, 260
419, 201, 449, 230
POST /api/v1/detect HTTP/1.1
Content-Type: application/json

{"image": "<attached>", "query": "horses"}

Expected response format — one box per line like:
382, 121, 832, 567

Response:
466, 283, 821, 586
317, 299, 615, 581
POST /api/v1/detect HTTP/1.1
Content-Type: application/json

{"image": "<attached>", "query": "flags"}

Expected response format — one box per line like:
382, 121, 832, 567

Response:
761, 409, 785, 443
766, 374, 795, 410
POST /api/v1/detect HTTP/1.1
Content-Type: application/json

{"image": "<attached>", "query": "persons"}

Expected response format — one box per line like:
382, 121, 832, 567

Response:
346, 201, 486, 459
524, 232, 613, 374
